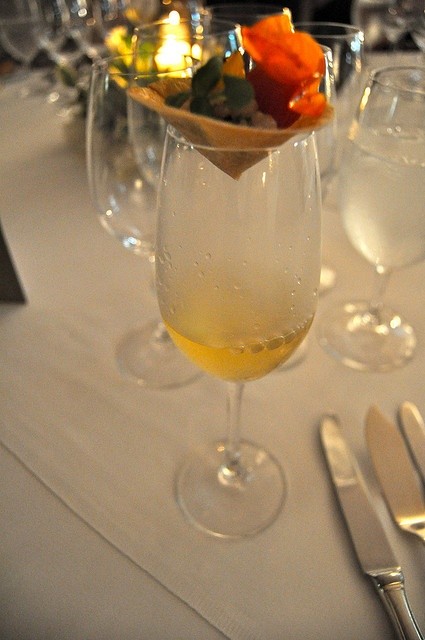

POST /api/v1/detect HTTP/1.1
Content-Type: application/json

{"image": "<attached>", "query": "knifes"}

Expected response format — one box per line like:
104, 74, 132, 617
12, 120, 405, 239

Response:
316, 413, 425, 640
363, 405, 425, 548
398, 399, 425, 484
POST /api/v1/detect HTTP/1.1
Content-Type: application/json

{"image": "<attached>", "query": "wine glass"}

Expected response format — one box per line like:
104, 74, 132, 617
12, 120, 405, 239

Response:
85, 54, 208, 391
292, 21, 364, 107
350, 1, 425, 61
1, 1, 208, 112
270, 41, 338, 188
314, 65, 425, 372
153, 125, 325, 539
126, 19, 251, 195
